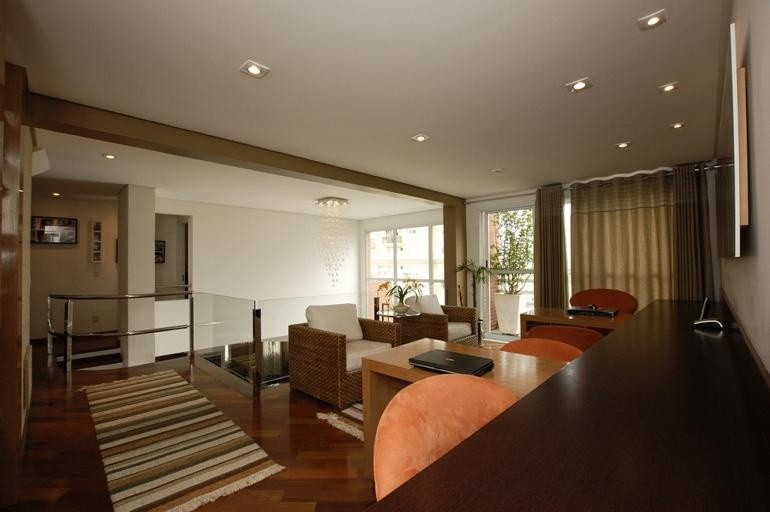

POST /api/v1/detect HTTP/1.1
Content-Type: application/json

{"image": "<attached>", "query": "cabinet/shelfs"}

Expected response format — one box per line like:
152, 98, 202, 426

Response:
89, 219, 104, 263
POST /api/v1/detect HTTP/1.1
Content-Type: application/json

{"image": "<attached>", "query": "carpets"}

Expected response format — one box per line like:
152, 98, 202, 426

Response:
84, 368, 286, 512
315, 403, 363, 442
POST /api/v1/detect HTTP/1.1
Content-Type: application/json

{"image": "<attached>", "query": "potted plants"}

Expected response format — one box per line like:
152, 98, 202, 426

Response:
455, 210, 534, 335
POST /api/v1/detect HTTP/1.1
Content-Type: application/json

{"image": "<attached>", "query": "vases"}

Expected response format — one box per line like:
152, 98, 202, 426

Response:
393, 297, 410, 317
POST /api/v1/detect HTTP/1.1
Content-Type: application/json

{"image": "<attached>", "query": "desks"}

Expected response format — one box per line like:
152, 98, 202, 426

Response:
520, 308, 632, 343
362, 338, 570, 447
358, 300, 770, 512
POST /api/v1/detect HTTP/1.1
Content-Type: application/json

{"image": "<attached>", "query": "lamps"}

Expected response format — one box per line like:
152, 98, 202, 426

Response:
315, 196, 352, 288
638, 9, 669, 32
237, 60, 269, 81
565, 76, 595, 95
656, 80, 678, 95
411, 132, 430, 143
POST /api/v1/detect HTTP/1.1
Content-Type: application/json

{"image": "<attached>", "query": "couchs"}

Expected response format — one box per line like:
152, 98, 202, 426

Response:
289, 303, 400, 408
393, 296, 479, 347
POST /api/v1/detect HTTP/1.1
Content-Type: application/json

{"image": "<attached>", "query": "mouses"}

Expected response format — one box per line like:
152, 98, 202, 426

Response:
588, 304, 597, 310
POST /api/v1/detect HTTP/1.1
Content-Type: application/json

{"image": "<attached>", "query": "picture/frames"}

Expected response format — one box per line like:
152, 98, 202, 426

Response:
30, 215, 78, 244
156, 240, 166, 265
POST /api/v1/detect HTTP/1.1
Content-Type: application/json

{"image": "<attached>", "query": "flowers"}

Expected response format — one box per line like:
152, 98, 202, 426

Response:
376, 279, 425, 304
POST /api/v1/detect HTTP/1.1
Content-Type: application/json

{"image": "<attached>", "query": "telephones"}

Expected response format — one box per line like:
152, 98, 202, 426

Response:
693, 297, 724, 329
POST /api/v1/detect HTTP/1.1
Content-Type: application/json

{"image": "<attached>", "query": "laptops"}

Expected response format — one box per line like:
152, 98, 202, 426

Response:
408, 348, 495, 377
567, 305, 619, 318
